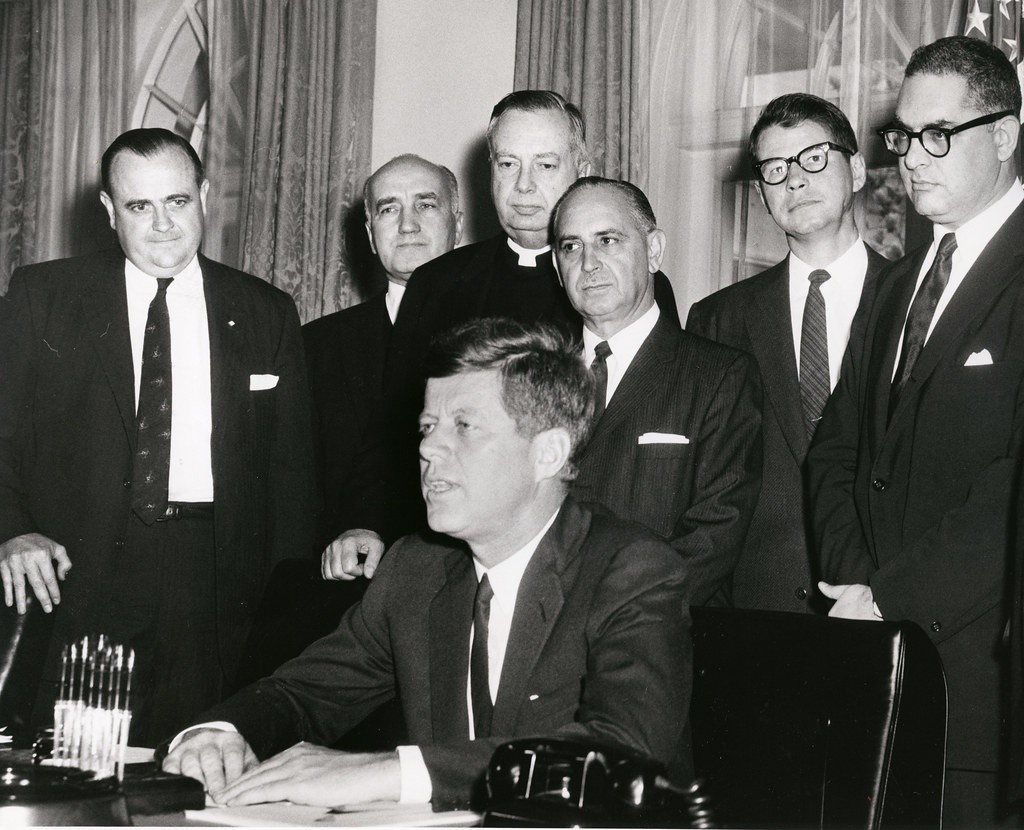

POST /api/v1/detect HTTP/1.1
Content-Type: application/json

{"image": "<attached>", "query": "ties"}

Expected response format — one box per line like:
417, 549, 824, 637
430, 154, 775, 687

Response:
471, 573, 495, 739
581, 339, 610, 441
887, 232, 958, 430
799, 268, 830, 442
130, 276, 171, 525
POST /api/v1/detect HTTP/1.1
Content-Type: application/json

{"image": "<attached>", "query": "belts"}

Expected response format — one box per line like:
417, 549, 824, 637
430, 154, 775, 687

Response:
156, 502, 214, 522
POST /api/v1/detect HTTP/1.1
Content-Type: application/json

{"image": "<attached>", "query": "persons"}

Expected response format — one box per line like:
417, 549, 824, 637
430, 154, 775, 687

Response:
554, 179, 766, 608
154, 316, 693, 812
301, 154, 463, 752
1, 126, 300, 748
684, 90, 892, 611
802, 34, 1023, 829
382, 88, 682, 545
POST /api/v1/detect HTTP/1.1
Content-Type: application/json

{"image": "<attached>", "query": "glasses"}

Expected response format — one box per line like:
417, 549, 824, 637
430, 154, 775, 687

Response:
874, 110, 1016, 158
755, 140, 856, 186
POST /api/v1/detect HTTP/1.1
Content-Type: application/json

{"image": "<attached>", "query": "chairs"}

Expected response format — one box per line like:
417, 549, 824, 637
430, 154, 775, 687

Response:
690, 605, 948, 830
